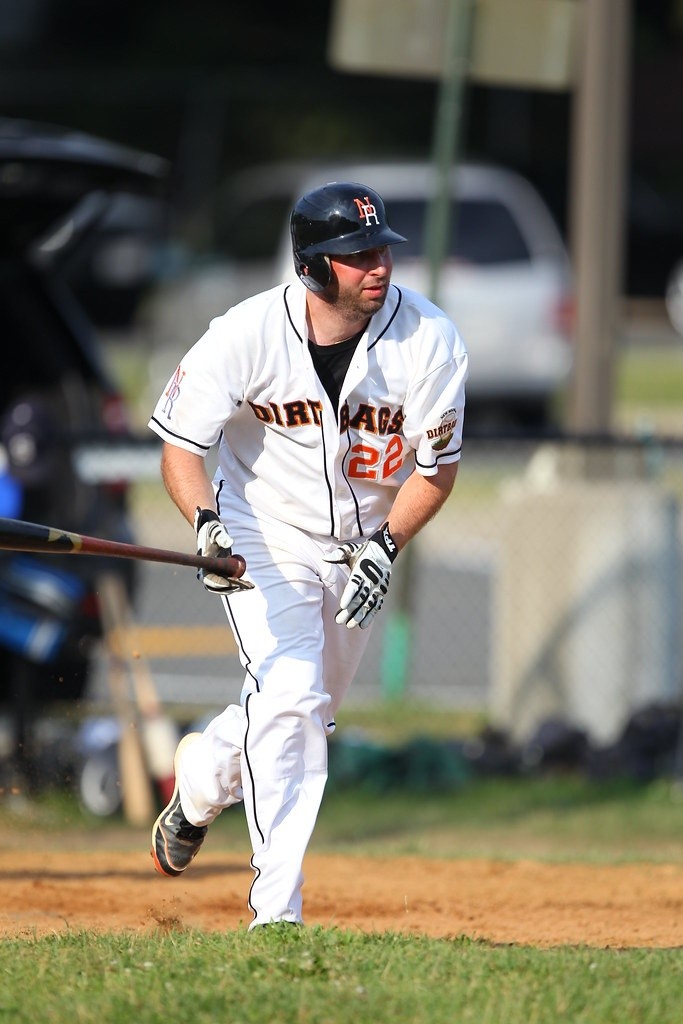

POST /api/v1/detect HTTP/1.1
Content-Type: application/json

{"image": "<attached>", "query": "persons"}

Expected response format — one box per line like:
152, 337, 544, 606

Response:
149, 178, 469, 931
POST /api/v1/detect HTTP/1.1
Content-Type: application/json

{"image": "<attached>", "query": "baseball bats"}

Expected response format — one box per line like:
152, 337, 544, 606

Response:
0, 517, 247, 580
105, 568, 183, 809
91, 568, 157, 829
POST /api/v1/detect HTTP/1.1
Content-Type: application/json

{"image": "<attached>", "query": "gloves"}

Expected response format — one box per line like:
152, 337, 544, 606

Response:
321, 520, 399, 631
193, 505, 255, 596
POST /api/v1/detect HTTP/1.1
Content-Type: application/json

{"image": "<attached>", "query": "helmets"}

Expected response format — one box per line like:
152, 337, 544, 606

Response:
289, 180, 407, 293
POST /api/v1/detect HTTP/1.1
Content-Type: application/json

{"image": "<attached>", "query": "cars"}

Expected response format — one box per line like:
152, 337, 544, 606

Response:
148, 158, 585, 440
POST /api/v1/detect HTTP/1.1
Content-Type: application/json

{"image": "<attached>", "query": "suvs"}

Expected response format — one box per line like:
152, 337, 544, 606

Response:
0, 120, 174, 727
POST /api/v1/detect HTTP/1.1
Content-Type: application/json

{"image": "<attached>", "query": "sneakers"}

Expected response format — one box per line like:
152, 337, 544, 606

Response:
150, 732, 208, 880
